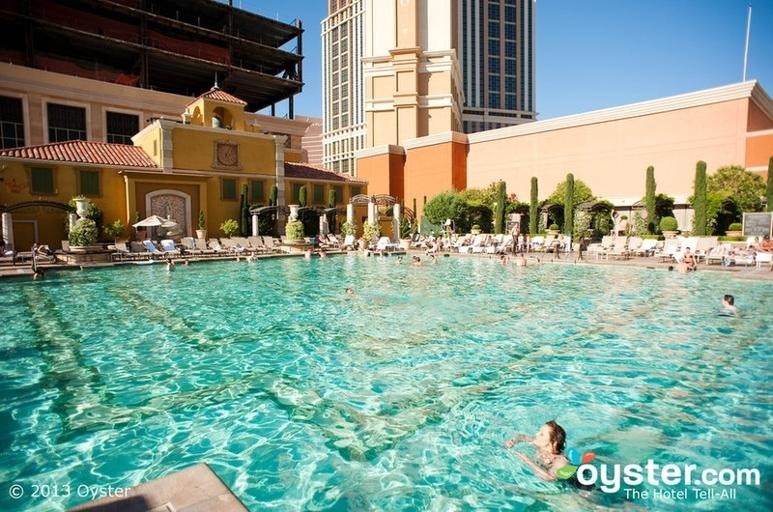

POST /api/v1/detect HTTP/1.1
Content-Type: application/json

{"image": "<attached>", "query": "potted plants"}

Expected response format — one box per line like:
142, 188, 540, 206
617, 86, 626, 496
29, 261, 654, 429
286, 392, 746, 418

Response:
194, 208, 207, 238
133, 210, 147, 240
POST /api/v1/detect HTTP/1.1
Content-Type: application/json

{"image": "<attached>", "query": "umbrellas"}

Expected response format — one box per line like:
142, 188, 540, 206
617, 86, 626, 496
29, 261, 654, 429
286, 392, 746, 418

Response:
131, 214, 177, 230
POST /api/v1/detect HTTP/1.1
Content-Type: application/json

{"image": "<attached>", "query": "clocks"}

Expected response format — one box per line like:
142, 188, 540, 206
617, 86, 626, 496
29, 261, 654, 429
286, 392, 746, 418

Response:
212, 139, 240, 169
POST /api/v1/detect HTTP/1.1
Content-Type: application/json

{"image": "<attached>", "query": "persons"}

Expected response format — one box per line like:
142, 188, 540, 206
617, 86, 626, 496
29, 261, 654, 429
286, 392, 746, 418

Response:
668, 247, 698, 272
722, 295, 737, 310
304, 222, 585, 268
166, 258, 189, 271
505, 421, 596, 490
32, 240, 49, 256
725, 235, 773, 271
0, 235, 5, 257
346, 288, 357, 295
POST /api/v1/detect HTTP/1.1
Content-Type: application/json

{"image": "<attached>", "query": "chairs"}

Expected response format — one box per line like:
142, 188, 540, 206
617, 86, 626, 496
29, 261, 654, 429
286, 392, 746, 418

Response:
457, 233, 772, 270
108, 234, 283, 262
